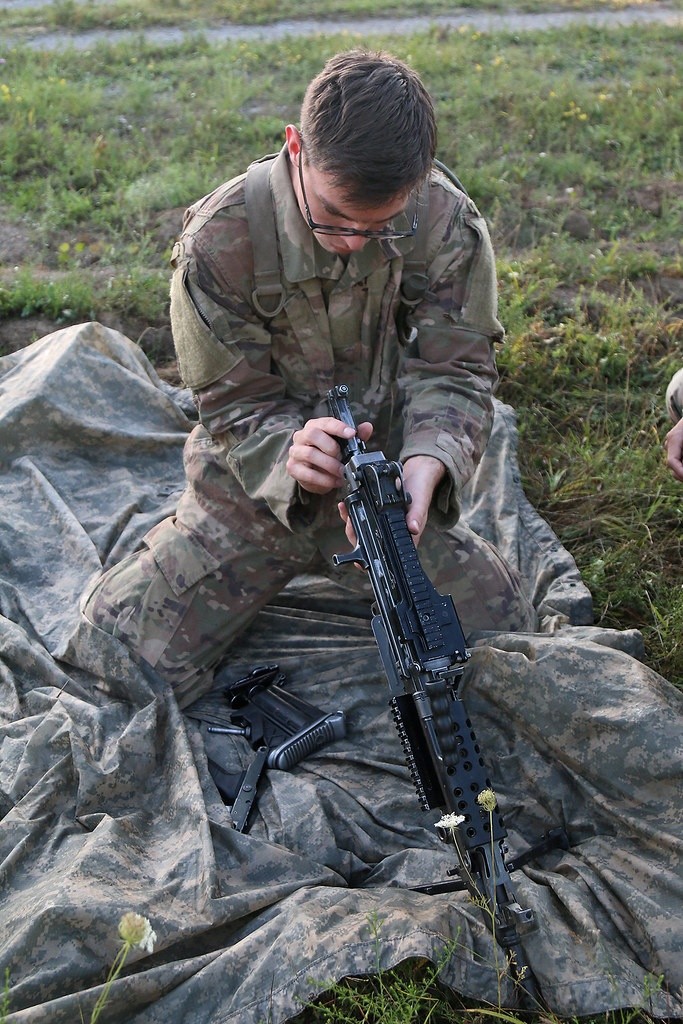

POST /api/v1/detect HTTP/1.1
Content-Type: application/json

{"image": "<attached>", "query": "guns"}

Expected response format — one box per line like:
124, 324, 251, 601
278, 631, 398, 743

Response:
321, 380, 575, 1016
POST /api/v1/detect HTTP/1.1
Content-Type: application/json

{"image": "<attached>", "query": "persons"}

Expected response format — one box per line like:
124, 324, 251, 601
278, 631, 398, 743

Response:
82, 51, 539, 709
660, 367, 682, 477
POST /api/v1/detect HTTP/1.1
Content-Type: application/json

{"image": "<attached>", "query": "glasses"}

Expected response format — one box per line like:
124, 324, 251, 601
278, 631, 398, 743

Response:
297, 139, 420, 239
